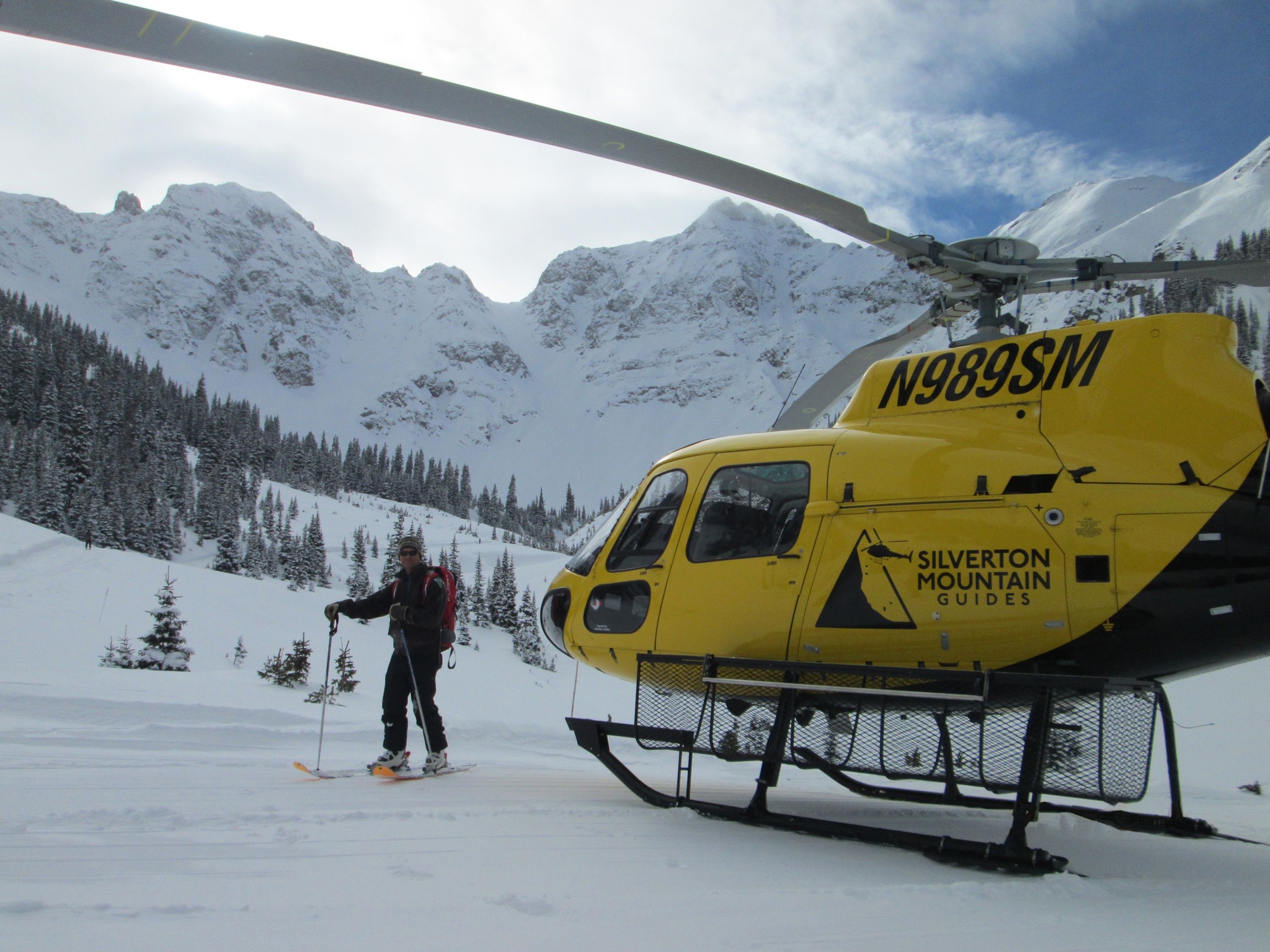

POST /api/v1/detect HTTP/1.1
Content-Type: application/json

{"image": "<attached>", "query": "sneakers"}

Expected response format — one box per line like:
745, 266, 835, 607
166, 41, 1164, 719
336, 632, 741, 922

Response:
378, 749, 406, 765
423, 750, 446, 771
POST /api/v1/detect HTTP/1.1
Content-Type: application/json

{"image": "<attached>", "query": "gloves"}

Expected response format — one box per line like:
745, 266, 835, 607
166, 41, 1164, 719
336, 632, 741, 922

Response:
325, 603, 340, 619
389, 602, 409, 621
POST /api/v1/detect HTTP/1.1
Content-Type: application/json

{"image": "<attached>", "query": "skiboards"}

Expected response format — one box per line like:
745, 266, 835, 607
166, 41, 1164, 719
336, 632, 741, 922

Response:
293, 757, 477, 781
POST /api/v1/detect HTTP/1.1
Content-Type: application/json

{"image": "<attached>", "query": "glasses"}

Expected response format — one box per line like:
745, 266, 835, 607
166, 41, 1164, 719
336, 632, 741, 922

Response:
399, 550, 419, 556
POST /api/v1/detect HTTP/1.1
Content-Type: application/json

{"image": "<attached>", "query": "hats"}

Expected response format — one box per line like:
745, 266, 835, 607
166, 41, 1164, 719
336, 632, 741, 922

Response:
398, 536, 422, 560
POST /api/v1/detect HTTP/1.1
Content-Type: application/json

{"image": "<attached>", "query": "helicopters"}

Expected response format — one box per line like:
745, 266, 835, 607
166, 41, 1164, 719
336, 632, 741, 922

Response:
0, 0, 1270, 880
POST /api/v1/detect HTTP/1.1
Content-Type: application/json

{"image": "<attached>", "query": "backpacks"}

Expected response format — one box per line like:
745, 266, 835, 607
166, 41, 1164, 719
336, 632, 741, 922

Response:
393, 566, 457, 652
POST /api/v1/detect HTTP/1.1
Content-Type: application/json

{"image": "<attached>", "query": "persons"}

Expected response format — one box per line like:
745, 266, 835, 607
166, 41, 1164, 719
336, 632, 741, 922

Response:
324, 536, 448, 772
